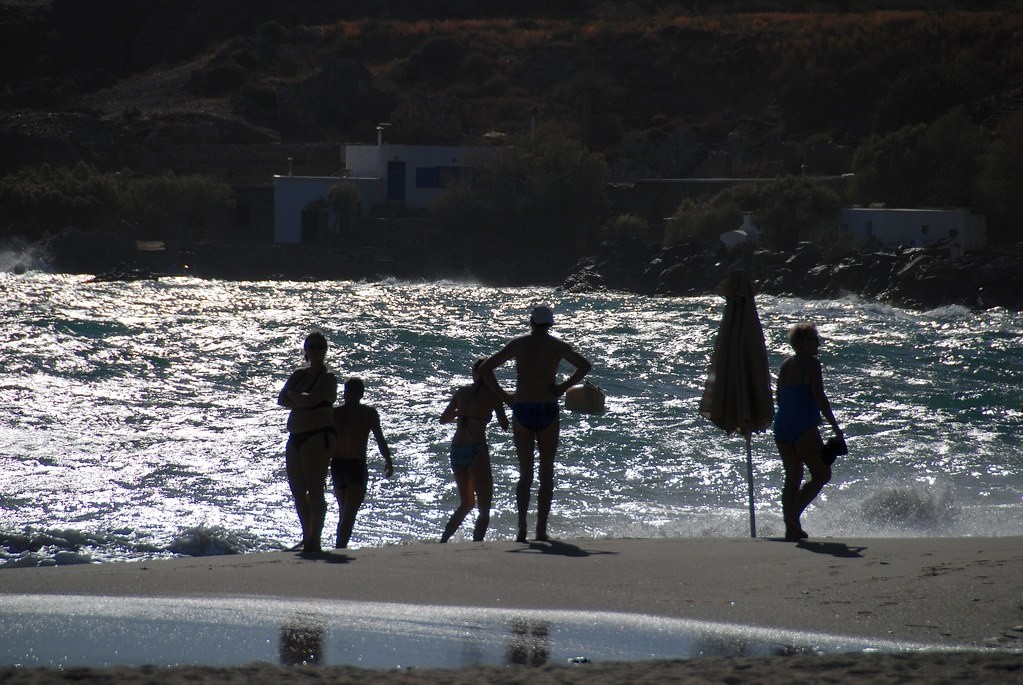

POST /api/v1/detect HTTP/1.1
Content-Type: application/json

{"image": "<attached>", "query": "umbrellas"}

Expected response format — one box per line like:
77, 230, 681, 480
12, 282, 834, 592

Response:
697, 245, 774, 537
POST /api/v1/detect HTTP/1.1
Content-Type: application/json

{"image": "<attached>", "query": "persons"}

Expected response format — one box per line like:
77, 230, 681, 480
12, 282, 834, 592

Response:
330, 376, 394, 548
437, 358, 509, 544
773, 322, 848, 540
278, 332, 337, 559
480, 304, 593, 545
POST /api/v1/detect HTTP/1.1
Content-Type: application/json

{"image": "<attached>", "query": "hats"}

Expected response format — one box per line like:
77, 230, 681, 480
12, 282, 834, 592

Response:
531, 304, 554, 324
